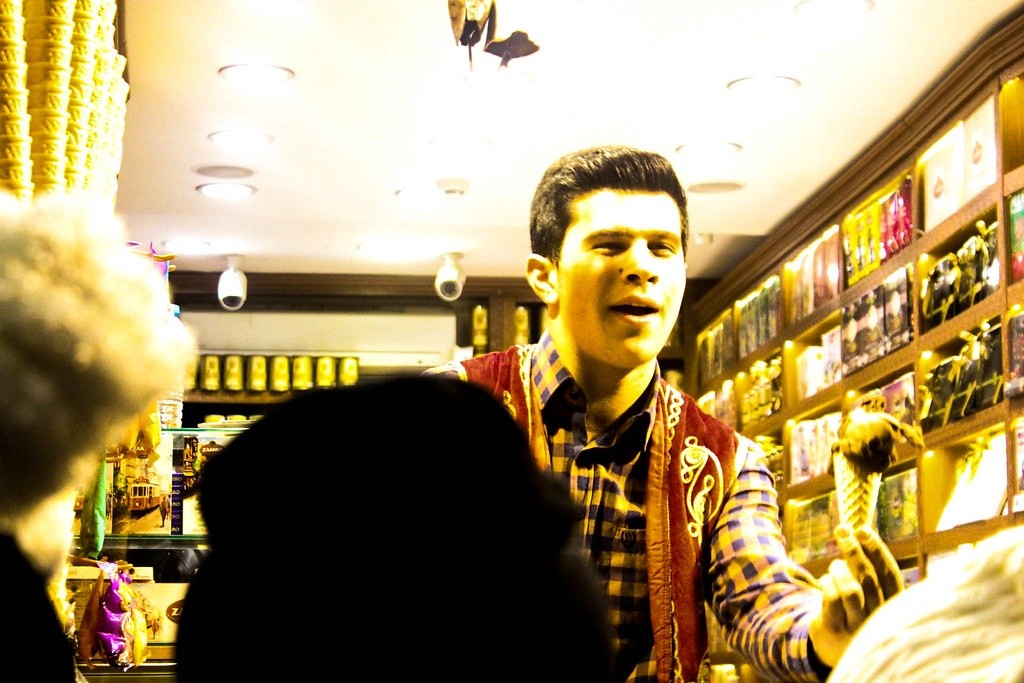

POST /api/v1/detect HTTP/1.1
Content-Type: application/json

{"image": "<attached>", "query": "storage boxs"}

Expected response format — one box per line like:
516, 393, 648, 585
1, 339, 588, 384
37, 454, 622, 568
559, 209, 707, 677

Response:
711, 328, 722, 379
812, 241, 825, 308
841, 301, 859, 378
767, 277, 779, 336
880, 372, 914, 425
859, 285, 885, 367
960, 93, 996, 206
883, 265, 910, 355
963, 344, 1005, 417
722, 312, 734, 371
821, 324, 841, 389
698, 333, 710, 385
923, 146, 965, 234
118, 580, 190, 644
795, 346, 821, 400
920, 356, 958, 433
747, 294, 758, 356
758, 287, 768, 346
947, 357, 980, 419
823, 232, 839, 305
738, 303, 749, 363
884, 467, 919, 537
786, 492, 831, 560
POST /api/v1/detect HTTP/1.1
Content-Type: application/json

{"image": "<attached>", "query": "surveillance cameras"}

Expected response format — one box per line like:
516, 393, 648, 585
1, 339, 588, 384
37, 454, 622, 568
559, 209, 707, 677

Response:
217, 269, 247, 310
435, 262, 467, 300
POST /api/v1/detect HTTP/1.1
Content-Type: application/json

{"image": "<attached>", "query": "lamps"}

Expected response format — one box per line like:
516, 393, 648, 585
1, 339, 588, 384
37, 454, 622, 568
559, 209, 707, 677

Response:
445, 0, 541, 83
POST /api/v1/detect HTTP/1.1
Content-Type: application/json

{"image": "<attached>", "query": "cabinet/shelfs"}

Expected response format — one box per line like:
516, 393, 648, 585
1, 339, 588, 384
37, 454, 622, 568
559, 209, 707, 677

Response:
53, 276, 684, 683
686, 1, 1024, 683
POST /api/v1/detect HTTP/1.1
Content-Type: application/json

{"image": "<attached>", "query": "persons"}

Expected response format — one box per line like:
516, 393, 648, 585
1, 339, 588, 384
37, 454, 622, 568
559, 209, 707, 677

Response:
419, 148, 907, 683
1, 191, 198, 683
174, 370, 626, 682
